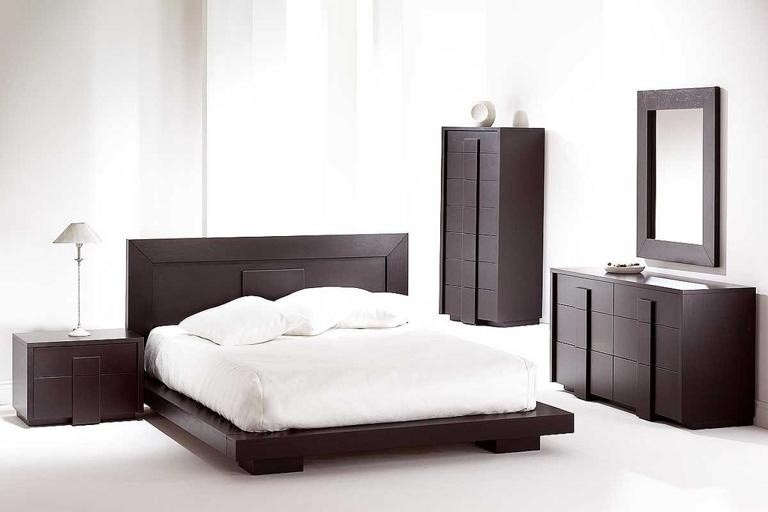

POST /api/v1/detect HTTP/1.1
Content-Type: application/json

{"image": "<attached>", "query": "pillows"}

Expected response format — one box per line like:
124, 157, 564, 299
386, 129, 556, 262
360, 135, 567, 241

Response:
272, 286, 366, 337
331, 292, 432, 330
178, 296, 300, 347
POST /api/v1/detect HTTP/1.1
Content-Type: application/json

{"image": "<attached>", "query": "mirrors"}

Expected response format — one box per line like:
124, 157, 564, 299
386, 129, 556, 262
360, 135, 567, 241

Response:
636, 85, 722, 267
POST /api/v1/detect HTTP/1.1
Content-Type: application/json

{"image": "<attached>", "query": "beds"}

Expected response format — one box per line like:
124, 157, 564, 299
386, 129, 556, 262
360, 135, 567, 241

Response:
127, 231, 575, 476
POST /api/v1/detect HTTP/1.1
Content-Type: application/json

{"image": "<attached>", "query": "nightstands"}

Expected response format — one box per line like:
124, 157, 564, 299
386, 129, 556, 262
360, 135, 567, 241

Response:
11, 328, 145, 428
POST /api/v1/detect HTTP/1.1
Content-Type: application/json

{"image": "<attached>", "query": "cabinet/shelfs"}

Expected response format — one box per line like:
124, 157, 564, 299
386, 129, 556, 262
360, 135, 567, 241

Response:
438, 127, 545, 328
549, 268, 756, 430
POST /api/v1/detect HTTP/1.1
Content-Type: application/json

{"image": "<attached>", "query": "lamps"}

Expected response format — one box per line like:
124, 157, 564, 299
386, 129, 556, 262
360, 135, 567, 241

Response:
53, 222, 103, 336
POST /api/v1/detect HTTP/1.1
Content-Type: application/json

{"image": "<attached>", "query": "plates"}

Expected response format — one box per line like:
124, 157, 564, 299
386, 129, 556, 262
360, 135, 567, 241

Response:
605, 265, 646, 274
